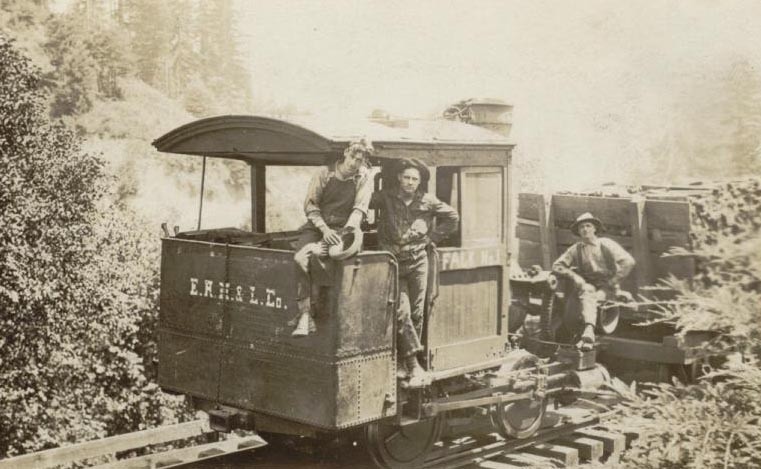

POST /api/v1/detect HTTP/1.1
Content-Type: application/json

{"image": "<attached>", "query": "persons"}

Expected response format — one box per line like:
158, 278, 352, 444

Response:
552, 212, 637, 344
287, 137, 375, 336
368, 158, 460, 385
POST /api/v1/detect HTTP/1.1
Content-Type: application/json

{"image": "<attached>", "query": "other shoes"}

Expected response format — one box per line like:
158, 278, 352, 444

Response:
581, 325, 595, 352
291, 316, 309, 337
287, 312, 316, 332
405, 357, 427, 382
395, 358, 409, 379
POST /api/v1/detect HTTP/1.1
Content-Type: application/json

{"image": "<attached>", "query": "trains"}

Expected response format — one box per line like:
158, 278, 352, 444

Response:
157, 107, 760, 469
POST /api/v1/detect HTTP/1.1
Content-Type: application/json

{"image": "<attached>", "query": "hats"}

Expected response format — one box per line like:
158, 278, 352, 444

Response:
569, 212, 601, 236
